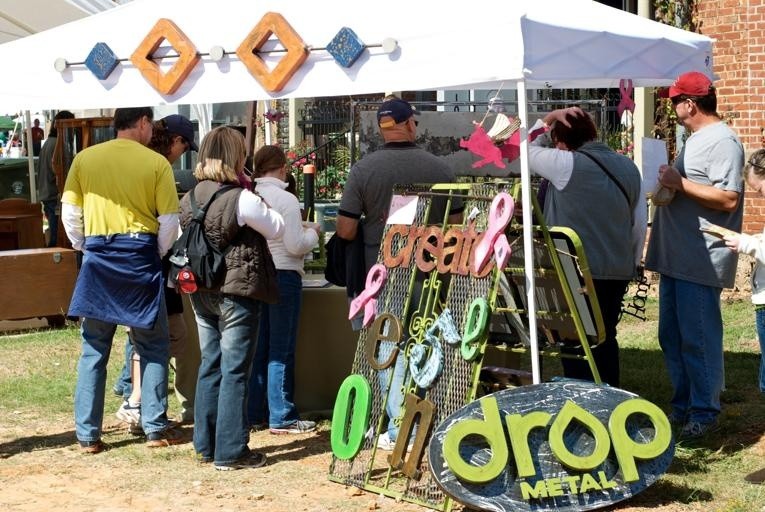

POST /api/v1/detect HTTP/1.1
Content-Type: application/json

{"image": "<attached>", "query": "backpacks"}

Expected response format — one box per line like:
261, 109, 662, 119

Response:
167, 185, 244, 294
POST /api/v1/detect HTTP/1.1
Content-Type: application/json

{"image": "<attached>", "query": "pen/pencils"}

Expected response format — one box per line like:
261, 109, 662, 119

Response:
307, 206, 312, 222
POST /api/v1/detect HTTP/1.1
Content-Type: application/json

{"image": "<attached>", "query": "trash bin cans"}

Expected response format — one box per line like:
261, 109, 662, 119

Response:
0, 158, 37, 203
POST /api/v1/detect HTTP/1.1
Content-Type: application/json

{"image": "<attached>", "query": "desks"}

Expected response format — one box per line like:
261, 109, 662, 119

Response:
0, 198, 45, 251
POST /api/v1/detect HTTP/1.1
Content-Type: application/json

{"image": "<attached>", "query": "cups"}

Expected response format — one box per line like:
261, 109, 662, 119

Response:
301, 221, 313, 233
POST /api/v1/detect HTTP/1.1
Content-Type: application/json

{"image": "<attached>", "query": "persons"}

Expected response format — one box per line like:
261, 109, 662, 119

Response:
1, 106, 321, 470
519, 103, 642, 389
335, 97, 467, 455
722, 148, 765, 484
643, 71, 747, 437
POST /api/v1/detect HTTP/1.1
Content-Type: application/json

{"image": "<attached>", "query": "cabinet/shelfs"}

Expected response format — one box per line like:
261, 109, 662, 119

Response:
51, 117, 114, 249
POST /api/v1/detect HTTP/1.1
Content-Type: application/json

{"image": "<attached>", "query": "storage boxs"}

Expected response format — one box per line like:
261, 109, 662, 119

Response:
0, 247, 78, 322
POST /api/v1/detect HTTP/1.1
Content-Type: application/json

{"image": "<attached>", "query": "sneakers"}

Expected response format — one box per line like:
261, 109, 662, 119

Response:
269, 417, 318, 436
667, 407, 688, 427
371, 430, 414, 453
115, 397, 141, 424
78, 437, 105, 454
145, 426, 193, 448
679, 415, 722, 440
126, 415, 178, 436
214, 449, 267, 472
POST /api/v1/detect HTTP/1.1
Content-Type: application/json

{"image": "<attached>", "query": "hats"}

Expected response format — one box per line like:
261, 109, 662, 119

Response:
163, 114, 199, 153
376, 98, 422, 129
656, 71, 718, 99
